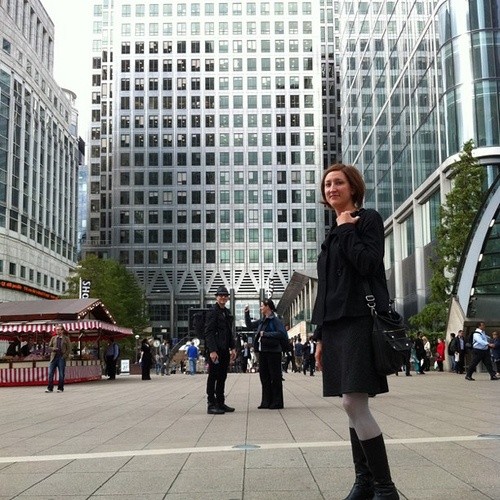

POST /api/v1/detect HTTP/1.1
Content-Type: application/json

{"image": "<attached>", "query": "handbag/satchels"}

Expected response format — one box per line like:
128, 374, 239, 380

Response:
372, 311, 412, 376
454, 353, 460, 361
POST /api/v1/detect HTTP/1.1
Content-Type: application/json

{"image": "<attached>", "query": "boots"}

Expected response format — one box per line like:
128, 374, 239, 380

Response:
359, 432, 400, 500
344, 427, 373, 500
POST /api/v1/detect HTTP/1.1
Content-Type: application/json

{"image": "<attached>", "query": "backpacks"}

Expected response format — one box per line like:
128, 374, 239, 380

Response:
465, 331, 482, 348
113, 343, 121, 357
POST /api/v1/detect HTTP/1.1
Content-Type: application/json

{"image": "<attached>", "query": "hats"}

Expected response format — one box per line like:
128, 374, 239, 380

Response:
215, 286, 231, 296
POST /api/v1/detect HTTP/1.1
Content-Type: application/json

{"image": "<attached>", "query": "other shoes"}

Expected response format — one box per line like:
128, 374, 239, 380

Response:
256, 403, 284, 409
57, 389, 63, 393
207, 405, 225, 414
44, 389, 53, 393
490, 374, 500, 380
464, 375, 476, 381
216, 402, 236, 412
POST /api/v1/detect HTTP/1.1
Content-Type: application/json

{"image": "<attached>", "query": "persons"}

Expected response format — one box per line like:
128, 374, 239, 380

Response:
245, 299, 285, 410
141, 339, 151, 380
447, 330, 467, 374
187, 342, 199, 375
203, 285, 237, 415
82, 345, 89, 354
465, 321, 500, 380
104, 337, 118, 380
489, 330, 500, 376
45, 325, 72, 393
237, 342, 258, 373
394, 331, 445, 376
170, 358, 185, 374
310, 163, 400, 500
6, 334, 34, 356
156, 337, 170, 376
285, 337, 316, 376
